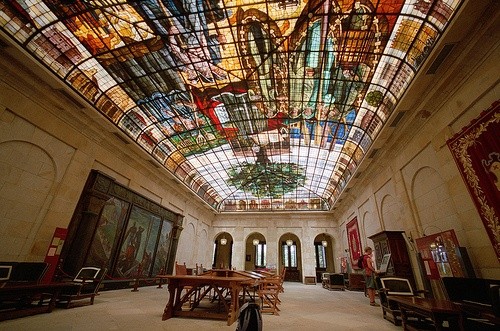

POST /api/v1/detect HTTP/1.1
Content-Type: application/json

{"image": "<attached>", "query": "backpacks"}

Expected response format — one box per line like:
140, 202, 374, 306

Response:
357, 254, 366, 270
235, 298, 262, 331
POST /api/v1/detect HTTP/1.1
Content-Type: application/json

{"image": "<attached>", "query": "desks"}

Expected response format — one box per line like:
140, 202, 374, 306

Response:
157, 274, 253, 326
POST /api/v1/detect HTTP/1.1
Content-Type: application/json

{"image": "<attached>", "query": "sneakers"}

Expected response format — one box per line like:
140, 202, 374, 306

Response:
369, 300, 380, 306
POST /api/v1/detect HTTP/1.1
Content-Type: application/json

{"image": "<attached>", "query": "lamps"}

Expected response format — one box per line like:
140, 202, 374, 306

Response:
221, 239, 227, 245
286, 240, 293, 246
253, 238, 259, 246
322, 240, 328, 247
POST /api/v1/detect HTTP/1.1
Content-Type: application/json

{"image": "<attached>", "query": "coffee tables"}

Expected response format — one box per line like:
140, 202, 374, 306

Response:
324, 279, 329, 290
385, 293, 474, 331
0, 281, 82, 322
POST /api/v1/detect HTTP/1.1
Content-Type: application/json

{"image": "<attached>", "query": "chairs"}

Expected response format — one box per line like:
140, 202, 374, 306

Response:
39, 265, 109, 309
196, 264, 212, 303
175, 261, 196, 308
328, 273, 346, 291
374, 275, 429, 327
213, 264, 230, 302
322, 273, 330, 287
256, 263, 286, 316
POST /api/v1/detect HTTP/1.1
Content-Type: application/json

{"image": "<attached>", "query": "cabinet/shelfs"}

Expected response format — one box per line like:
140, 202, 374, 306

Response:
368, 231, 417, 293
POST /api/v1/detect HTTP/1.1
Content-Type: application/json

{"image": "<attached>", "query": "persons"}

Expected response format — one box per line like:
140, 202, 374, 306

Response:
362, 247, 380, 307
